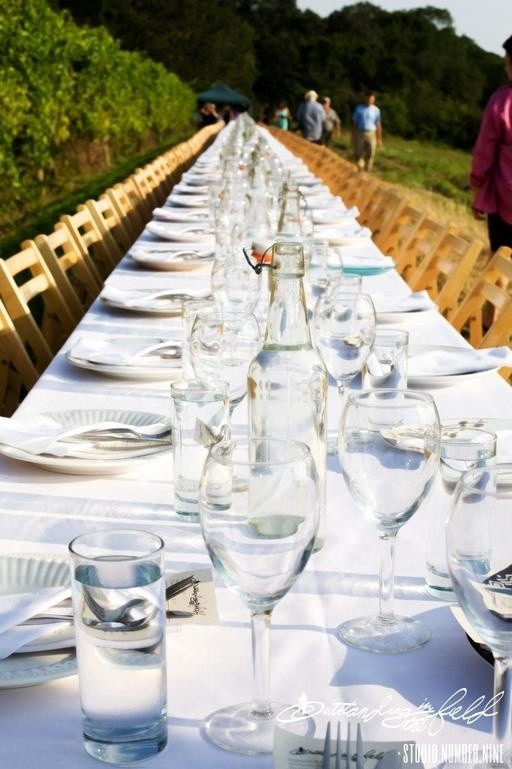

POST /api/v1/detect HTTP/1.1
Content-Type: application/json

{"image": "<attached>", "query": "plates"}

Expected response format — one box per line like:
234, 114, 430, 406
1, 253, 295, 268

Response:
0, 125, 512, 690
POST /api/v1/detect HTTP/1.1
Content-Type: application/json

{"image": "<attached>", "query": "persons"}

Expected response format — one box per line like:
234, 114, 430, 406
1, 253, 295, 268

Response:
200, 103, 233, 129
320, 96, 342, 149
350, 92, 382, 174
274, 101, 292, 131
470, 35, 512, 261
295, 88, 328, 145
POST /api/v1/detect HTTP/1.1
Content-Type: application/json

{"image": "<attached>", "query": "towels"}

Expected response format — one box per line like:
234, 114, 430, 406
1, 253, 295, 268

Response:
0, 419, 171, 457
0, 587, 74, 659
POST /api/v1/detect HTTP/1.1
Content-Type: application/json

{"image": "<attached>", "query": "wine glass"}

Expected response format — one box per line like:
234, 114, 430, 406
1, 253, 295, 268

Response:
191, 114, 512, 769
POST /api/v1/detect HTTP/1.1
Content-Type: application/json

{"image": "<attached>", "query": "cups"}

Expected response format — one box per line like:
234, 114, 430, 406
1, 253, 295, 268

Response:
68, 528, 168, 765
